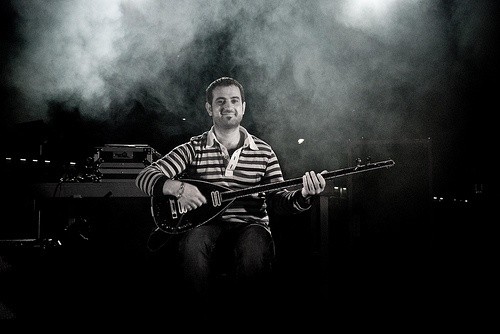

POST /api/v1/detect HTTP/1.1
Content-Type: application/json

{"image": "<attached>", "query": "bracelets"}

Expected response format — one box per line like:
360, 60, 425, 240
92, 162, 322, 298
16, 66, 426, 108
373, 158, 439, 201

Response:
177, 181, 184, 199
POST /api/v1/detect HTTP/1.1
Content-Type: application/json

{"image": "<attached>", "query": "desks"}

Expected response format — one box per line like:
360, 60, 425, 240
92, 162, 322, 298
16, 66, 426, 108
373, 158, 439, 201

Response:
34, 179, 151, 240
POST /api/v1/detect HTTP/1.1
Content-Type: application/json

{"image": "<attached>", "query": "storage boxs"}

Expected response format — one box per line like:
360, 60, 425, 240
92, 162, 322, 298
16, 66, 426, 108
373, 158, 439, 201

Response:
93, 143, 163, 175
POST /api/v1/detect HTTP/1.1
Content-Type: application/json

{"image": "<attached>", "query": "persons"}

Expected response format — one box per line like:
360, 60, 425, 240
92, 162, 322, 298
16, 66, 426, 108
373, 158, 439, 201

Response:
134, 77, 329, 334
